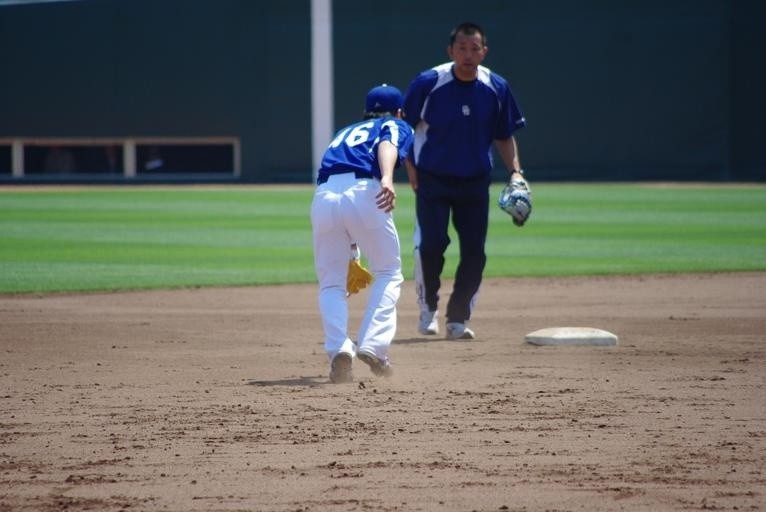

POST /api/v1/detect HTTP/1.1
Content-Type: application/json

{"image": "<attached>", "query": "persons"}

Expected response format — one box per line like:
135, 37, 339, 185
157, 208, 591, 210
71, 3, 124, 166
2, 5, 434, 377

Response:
311, 81, 419, 382
398, 20, 532, 341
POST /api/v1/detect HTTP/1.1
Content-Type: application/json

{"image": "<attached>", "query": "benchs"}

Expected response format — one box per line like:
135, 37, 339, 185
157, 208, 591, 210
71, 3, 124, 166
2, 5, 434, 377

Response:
0, 136, 241, 178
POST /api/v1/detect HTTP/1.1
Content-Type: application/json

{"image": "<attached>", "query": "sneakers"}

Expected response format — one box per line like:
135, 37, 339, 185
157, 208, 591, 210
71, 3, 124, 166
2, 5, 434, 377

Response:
444, 323, 474, 339
418, 311, 438, 336
329, 352, 354, 383
357, 350, 394, 378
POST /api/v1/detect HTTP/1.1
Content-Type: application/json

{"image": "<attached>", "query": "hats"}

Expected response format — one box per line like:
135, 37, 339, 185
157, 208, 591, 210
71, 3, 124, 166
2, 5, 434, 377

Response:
366, 83, 405, 112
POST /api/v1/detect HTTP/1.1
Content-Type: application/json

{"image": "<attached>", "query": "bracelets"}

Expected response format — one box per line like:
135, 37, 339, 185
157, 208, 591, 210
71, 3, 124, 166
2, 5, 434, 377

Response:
510, 168, 524, 175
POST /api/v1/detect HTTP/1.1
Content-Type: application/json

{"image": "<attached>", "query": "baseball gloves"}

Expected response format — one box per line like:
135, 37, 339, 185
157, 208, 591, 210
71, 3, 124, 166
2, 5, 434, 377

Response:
498, 182, 532, 228
347, 260, 371, 296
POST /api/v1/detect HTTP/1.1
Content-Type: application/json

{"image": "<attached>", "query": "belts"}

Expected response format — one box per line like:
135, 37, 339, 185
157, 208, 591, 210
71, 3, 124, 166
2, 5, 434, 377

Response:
320, 171, 373, 183
